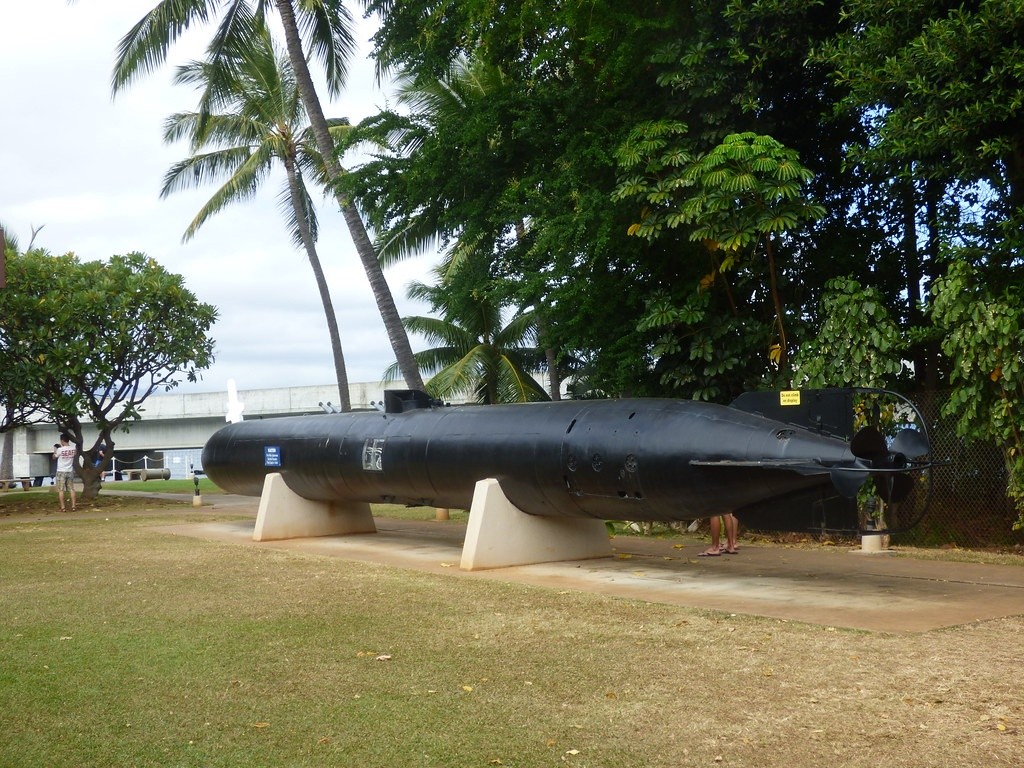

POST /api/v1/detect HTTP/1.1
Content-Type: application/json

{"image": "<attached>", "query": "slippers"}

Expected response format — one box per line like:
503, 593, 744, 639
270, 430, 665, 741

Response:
697, 549, 720, 556
719, 543, 739, 550
722, 549, 737, 554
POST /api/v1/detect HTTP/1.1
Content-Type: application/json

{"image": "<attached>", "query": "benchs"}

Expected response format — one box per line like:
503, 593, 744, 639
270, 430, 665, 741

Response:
0, 478, 36, 492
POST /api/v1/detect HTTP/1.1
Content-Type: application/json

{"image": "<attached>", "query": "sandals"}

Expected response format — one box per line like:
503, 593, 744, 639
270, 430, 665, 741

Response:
71, 507, 78, 512
56, 507, 66, 512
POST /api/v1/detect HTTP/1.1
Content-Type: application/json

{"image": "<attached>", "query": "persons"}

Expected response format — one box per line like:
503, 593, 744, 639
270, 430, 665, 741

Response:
697, 512, 747, 557
52, 432, 78, 512
95, 441, 105, 482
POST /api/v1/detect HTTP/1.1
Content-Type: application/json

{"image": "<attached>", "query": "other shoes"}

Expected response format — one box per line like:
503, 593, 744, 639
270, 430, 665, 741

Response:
101, 479, 105, 482
